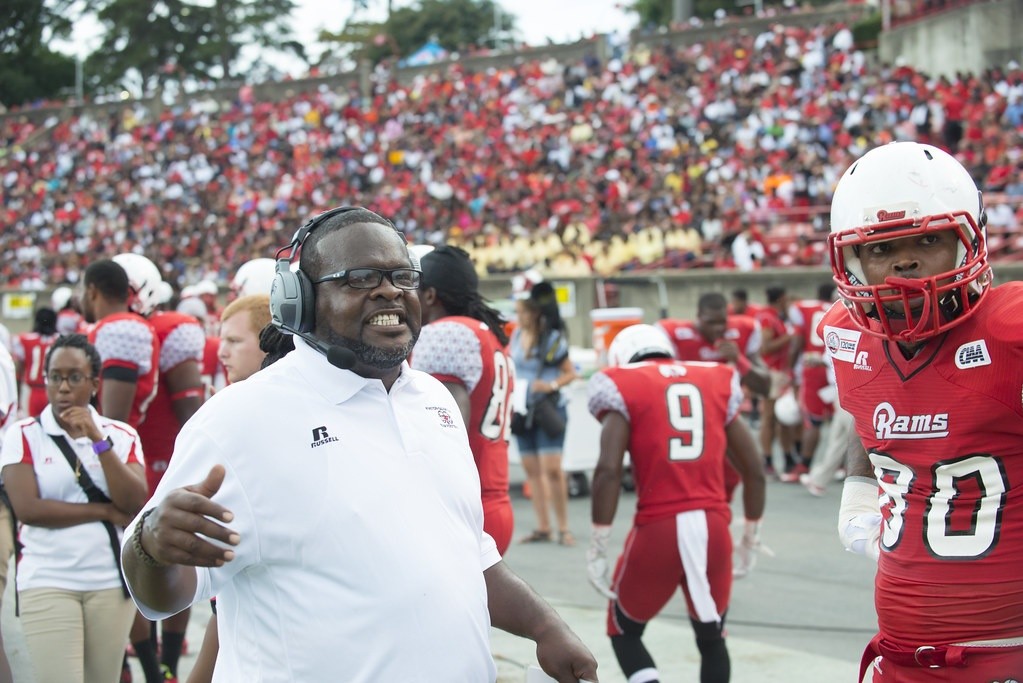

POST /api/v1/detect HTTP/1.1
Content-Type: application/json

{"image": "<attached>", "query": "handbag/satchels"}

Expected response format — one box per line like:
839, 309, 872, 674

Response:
537, 394, 563, 438
510, 403, 538, 432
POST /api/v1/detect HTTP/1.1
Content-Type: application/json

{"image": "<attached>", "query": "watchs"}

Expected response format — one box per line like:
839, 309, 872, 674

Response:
92, 436, 113, 454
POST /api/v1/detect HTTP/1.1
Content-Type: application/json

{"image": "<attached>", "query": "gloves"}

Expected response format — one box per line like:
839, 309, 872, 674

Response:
587, 524, 617, 599
732, 516, 774, 575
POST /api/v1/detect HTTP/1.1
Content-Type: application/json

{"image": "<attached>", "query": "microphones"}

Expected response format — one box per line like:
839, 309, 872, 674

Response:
270, 319, 358, 369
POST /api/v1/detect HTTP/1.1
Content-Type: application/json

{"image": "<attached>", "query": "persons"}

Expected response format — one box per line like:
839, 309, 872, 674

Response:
409, 246, 513, 555
664, 282, 845, 497
589, 324, 767, 683
507, 284, 578, 548
0, 1, 1022, 288
817, 139, 1023, 683
119, 205, 597, 683
0, 253, 284, 683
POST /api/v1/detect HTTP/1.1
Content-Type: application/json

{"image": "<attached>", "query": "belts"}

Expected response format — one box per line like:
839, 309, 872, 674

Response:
858, 635, 1022, 682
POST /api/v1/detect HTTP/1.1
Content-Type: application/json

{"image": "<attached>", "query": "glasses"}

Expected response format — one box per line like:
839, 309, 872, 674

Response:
42, 373, 93, 386
312, 266, 422, 290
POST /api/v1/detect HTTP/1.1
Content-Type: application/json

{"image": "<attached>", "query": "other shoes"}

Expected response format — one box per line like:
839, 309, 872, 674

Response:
124, 640, 188, 655
799, 472, 823, 496
558, 530, 577, 545
518, 530, 551, 542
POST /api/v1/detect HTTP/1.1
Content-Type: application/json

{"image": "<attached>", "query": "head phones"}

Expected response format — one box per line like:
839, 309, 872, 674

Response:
270, 204, 422, 335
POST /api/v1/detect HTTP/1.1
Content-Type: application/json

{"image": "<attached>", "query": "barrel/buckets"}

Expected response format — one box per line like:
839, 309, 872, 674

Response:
591, 306, 645, 363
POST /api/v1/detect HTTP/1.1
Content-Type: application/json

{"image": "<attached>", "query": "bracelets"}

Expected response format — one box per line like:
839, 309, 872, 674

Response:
551, 382, 558, 390
132, 507, 163, 568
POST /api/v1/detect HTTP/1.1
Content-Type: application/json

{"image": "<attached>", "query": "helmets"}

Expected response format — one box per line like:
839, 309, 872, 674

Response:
176, 297, 206, 320
827, 140, 992, 342
606, 324, 674, 367
227, 258, 276, 299
194, 280, 217, 296
51, 287, 73, 310
113, 252, 162, 316
